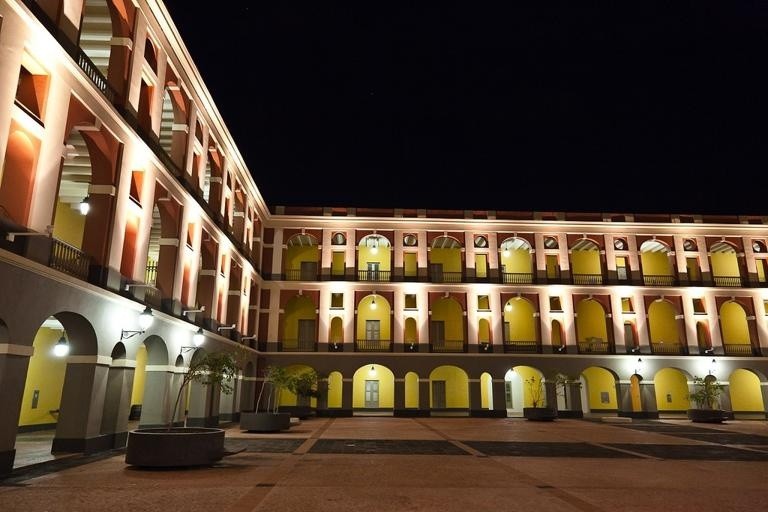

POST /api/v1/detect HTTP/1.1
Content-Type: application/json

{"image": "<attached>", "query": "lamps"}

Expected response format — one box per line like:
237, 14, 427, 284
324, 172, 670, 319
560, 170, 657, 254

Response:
506, 302, 512, 312
125, 284, 158, 291
183, 306, 205, 316
370, 296, 377, 310
369, 367, 376, 376
54, 329, 69, 357
80, 196, 90, 215
218, 324, 236, 331
242, 335, 257, 341
121, 304, 154, 340
503, 241, 510, 257
180, 327, 205, 354
371, 237, 378, 254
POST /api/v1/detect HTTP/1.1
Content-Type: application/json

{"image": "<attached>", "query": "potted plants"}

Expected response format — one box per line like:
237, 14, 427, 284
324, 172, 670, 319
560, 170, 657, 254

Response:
686, 375, 727, 423
240, 365, 291, 433
523, 367, 583, 422
125, 341, 253, 470
